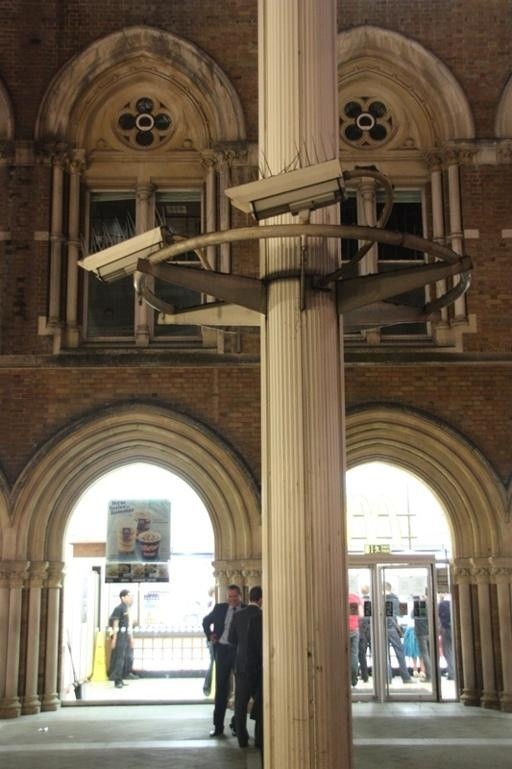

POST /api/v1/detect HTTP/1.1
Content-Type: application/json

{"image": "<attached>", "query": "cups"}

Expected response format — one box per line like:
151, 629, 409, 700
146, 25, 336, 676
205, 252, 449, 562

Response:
134, 511, 153, 533
116, 519, 137, 552
138, 531, 162, 561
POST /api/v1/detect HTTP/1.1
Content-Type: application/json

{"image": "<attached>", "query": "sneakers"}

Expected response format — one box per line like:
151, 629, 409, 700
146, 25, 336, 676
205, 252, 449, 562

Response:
115, 680, 129, 688
403, 670, 431, 683
209, 726, 263, 748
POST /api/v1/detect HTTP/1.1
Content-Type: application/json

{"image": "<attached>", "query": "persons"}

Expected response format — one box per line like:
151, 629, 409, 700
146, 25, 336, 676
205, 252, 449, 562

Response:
202, 585, 216, 696
346, 578, 454, 688
199, 583, 249, 739
106, 588, 143, 690
126, 595, 141, 680
225, 585, 262, 750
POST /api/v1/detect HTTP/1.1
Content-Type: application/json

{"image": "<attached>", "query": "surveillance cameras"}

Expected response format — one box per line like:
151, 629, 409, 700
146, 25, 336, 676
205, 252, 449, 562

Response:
224, 156, 350, 222
76, 224, 175, 287
